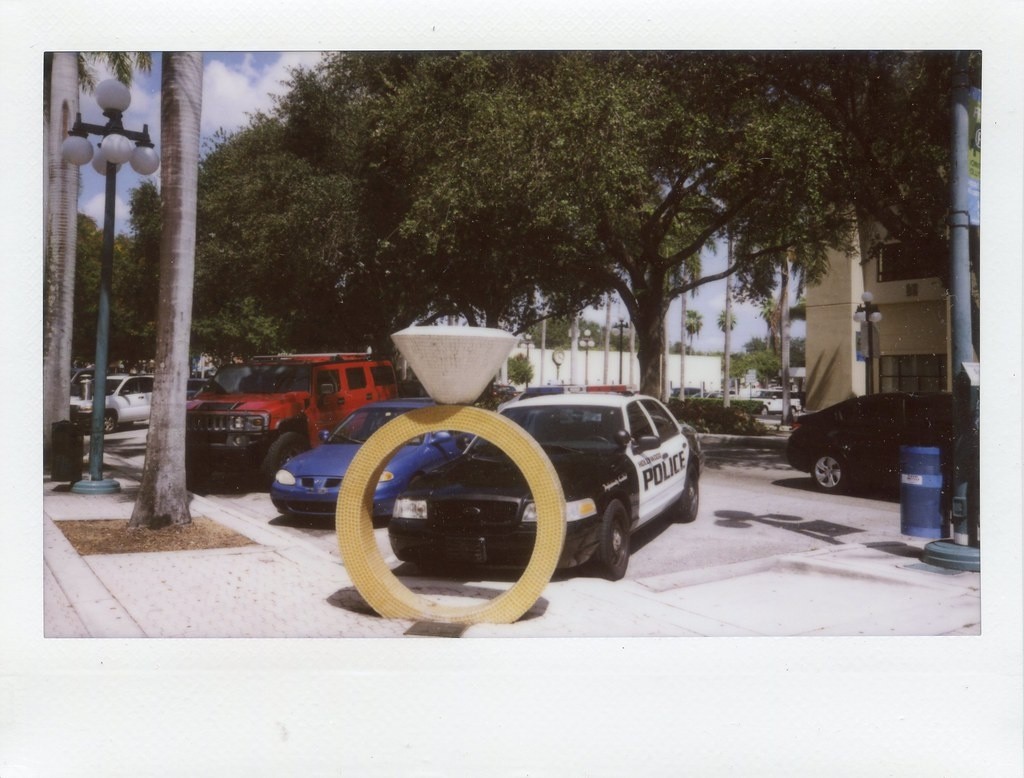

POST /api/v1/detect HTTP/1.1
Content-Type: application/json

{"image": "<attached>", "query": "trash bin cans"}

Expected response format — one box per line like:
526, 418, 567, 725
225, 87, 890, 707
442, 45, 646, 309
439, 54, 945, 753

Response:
48, 419, 84, 483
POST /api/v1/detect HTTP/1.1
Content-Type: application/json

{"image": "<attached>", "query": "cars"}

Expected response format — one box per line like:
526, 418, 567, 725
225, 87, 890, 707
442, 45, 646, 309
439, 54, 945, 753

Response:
68, 375, 162, 434
671, 387, 801, 418
784, 390, 980, 501
270, 399, 485, 526
389, 384, 704, 584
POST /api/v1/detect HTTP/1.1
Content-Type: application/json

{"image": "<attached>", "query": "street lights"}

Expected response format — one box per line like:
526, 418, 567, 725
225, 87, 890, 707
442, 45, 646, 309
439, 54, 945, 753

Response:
853, 292, 883, 394
59, 77, 160, 494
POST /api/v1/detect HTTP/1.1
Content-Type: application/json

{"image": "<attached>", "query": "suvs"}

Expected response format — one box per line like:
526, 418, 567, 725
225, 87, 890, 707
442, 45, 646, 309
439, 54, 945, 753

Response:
185, 351, 397, 493
